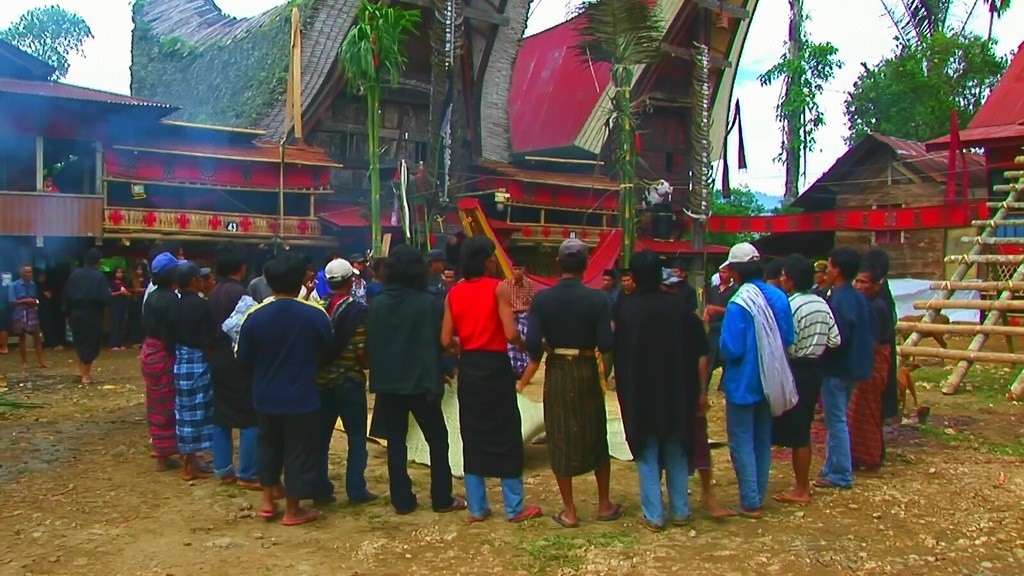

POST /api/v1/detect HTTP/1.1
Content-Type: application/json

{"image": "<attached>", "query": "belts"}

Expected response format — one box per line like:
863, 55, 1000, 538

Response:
550, 347, 596, 357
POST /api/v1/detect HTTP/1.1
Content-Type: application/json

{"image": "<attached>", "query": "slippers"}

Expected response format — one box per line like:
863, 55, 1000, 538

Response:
597, 503, 624, 522
552, 507, 580, 528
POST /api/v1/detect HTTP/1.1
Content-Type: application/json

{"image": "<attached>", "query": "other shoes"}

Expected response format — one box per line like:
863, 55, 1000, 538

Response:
280, 511, 326, 526
510, 505, 544, 521
434, 495, 469, 512
730, 502, 762, 518
467, 509, 493, 523
671, 512, 694, 525
813, 478, 854, 489
259, 504, 279, 516
771, 485, 815, 505
641, 512, 665, 532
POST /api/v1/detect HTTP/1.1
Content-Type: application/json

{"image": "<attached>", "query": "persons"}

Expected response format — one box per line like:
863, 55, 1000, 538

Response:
43, 174, 60, 192
108, 251, 460, 525
502, 259, 536, 380
439, 236, 542, 521
523, 237, 628, 526
0, 263, 73, 354
612, 249, 697, 531
597, 245, 898, 515
8, 264, 51, 368
365, 248, 470, 514
718, 243, 795, 518
59, 246, 111, 383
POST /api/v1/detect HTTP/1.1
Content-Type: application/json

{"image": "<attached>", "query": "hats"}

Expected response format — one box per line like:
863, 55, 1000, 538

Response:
559, 238, 588, 256
813, 260, 828, 272
151, 252, 188, 274
178, 261, 211, 280
324, 259, 360, 281
719, 241, 759, 270
